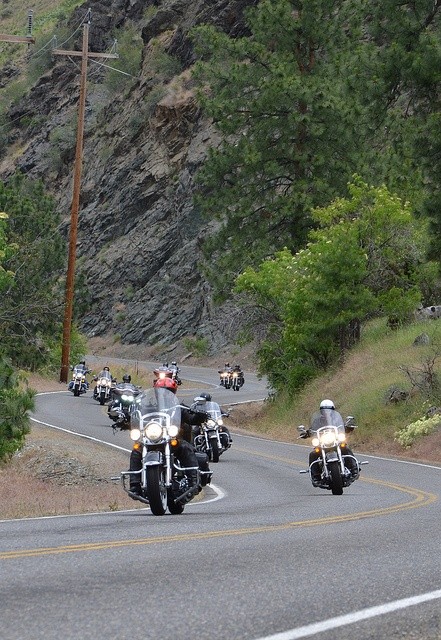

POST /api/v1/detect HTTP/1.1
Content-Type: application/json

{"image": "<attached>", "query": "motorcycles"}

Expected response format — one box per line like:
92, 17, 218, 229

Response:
168, 361, 181, 385
297, 399, 369, 495
121, 377, 211, 515
191, 393, 233, 462
152, 363, 178, 396
106, 374, 142, 430
231, 364, 244, 391
68, 361, 92, 396
91, 367, 117, 405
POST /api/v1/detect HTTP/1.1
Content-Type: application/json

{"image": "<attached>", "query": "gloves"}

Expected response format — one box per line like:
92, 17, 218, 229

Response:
300, 429, 312, 439
347, 423, 355, 433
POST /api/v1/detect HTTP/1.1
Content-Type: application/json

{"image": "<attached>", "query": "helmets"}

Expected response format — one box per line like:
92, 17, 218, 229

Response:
154, 377, 178, 388
172, 361, 177, 364
163, 362, 168, 366
104, 367, 109, 370
123, 374, 131, 380
320, 399, 335, 410
80, 360, 85, 363
225, 362, 229, 366
235, 364, 240, 367
200, 393, 211, 400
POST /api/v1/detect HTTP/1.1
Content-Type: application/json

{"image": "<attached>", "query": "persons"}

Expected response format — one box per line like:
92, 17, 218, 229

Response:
299, 399, 361, 482
154, 362, 168, 385
116, 377, 207, 500
94, 366, 115, 396
171, 361, 181, 385
68, 360, 90, 389
191, 393, 233, 447
233, 365, 244, 384
108, 374, 131, 416
